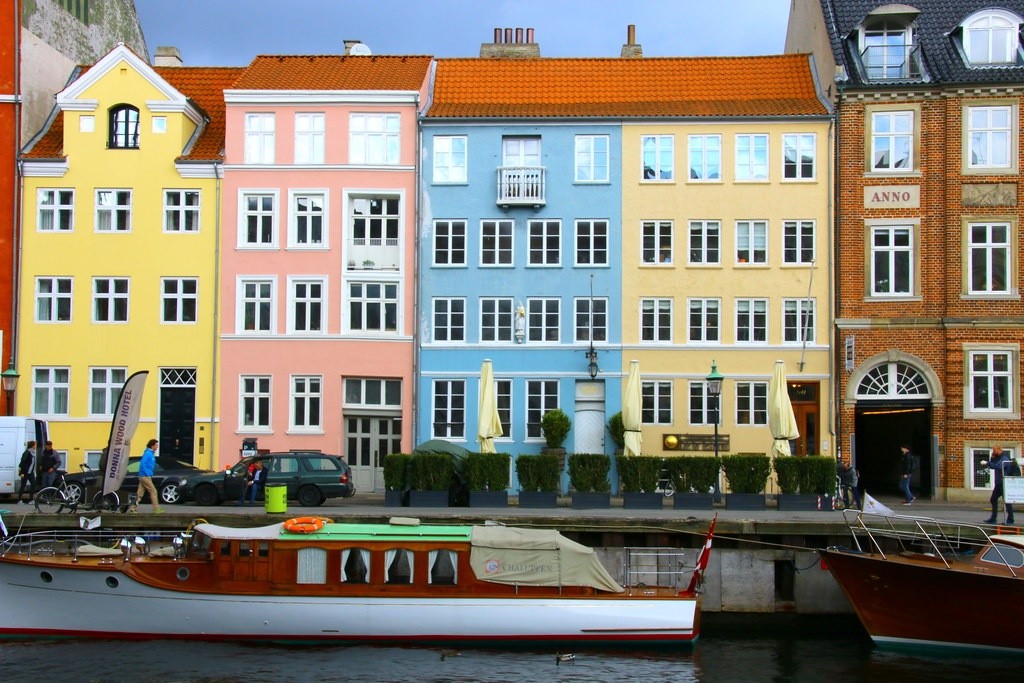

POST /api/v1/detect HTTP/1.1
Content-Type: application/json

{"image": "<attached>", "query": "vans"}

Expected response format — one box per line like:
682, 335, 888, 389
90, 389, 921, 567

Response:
1, 416, 49, 500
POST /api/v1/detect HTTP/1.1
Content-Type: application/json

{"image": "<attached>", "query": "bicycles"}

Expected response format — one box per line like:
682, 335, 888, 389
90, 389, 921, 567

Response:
834, 476, 854, 510
35, 464, 120, 513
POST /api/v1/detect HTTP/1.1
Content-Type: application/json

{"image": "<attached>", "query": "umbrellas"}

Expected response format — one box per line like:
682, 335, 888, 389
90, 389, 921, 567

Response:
623, 360, 642, 487
767, 360, 800, 511
477, 359, 503, 491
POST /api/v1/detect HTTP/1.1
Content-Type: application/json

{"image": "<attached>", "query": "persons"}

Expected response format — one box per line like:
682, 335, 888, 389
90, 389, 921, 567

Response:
980, 441, 1015, 525
135, 439, 166, 513
238, 460, 266, 507
166, 392, 189, 443
16, 441, 37, 504
857, 464, 869, 495
40, 441, 61, 502
843, 462, 861, 509
900, 443, 916, 503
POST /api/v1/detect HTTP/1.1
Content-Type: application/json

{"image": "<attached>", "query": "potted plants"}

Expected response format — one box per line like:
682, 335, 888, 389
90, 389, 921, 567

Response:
382, 412, 836, 512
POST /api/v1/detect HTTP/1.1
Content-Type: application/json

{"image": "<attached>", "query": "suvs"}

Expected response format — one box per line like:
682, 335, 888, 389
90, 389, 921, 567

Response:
182, 449, 357, 509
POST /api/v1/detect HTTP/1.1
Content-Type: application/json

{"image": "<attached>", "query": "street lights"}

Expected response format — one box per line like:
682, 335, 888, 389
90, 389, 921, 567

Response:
0, 356, 22, 416
704, 359, 722, 503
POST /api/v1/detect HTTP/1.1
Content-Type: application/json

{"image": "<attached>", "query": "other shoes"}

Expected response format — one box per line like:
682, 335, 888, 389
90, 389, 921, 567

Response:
902, 502, 911, 505
18, 501, 24, 505
152, 509, 164, 514
29, 500, 36, 504
128, 509, 139, 514
910, 496, 916, 503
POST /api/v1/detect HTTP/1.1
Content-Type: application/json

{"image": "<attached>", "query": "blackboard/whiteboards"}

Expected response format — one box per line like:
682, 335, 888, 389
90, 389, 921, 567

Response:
1001, 461, 1024, 505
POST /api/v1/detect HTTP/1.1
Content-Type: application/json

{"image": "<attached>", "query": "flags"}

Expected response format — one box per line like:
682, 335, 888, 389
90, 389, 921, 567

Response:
678, 512, 718, 597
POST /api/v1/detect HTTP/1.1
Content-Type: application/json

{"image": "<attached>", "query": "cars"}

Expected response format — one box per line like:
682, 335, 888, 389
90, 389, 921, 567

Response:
50, 453, 215, 506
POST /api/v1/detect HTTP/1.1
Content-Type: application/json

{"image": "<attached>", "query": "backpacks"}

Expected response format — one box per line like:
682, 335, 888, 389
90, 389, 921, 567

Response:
1000, 454, 1021, 476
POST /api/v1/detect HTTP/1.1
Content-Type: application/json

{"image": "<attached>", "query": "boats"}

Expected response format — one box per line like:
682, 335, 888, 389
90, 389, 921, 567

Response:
817, 508, 1024, 667
0, 511, 708, 653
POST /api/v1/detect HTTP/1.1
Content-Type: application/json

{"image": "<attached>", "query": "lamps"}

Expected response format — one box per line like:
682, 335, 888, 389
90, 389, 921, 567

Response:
583, 342, 601, 381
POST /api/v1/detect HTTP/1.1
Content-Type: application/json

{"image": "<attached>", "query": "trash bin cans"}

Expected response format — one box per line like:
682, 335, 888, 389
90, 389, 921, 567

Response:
265, 482, 288, 514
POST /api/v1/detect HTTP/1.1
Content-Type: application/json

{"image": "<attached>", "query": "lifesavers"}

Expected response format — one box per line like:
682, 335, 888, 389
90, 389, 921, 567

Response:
284, 517, 323, 534
311, 515, 334, 523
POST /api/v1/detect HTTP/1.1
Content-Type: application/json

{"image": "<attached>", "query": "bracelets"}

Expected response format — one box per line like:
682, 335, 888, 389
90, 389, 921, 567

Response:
252, 481, 254, 483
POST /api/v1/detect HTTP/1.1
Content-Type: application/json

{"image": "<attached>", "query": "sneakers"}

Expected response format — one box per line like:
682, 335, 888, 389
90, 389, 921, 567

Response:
983, 518, 997, 523
1002, 520, 1014, 525
